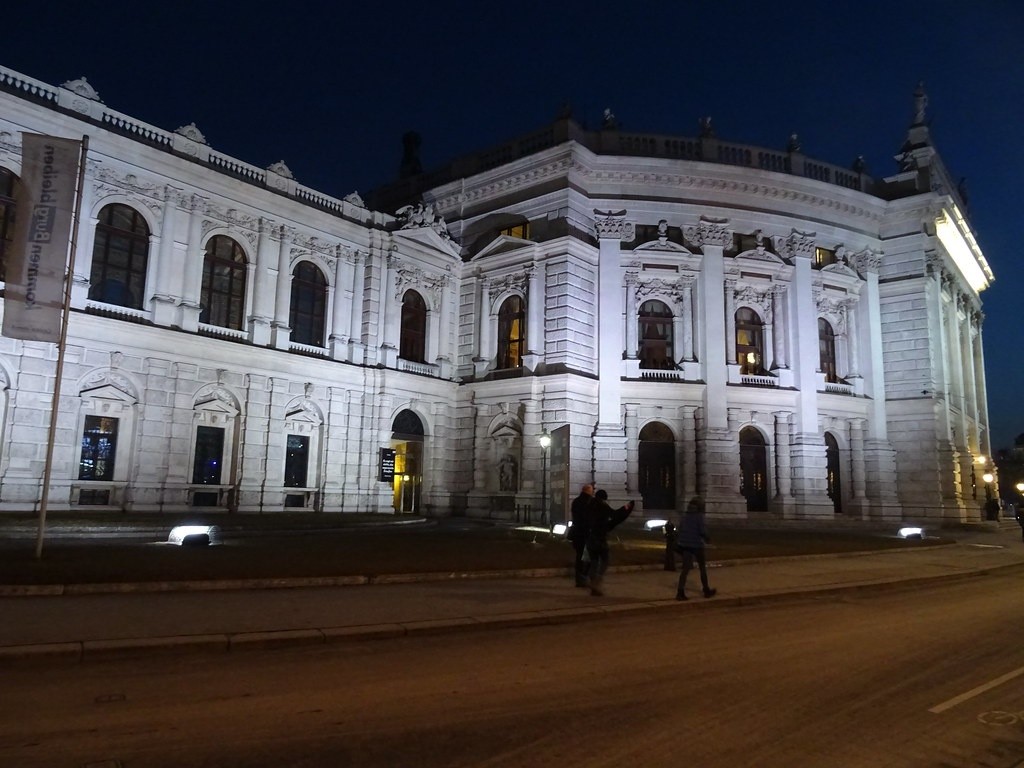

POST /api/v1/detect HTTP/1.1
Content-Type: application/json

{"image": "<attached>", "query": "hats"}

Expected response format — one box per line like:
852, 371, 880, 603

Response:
596, 490, 607, 500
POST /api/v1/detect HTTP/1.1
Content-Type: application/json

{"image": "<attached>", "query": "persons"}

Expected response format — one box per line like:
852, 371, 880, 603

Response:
675, 496, 716, 601
570, 485, 632, 596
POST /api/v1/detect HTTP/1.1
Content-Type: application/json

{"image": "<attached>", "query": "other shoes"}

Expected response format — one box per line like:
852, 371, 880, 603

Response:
675, 593, 688, 601
590, 588, 604, 598
703, 589, 717, 599
576, 581, 590, 587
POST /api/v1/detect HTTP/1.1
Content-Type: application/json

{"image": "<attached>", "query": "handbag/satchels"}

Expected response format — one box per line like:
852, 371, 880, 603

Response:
567, 520, 578, 540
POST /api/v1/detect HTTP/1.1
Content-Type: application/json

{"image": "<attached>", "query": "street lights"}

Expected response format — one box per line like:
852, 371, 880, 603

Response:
539, 428, 552, 525
